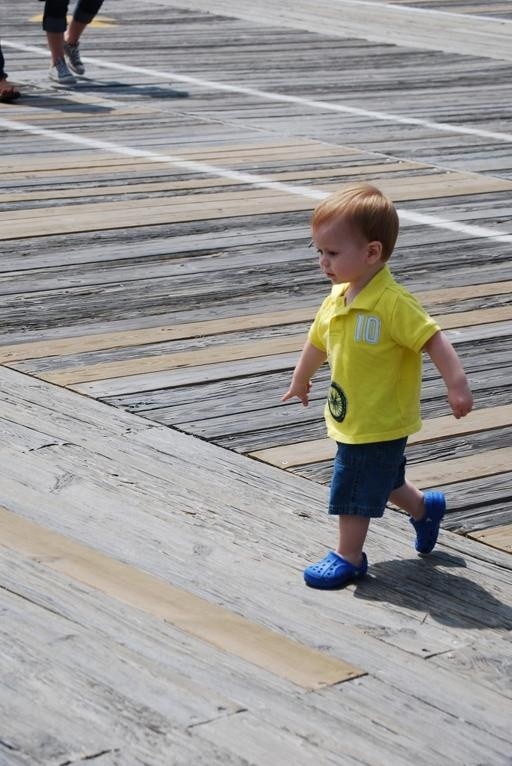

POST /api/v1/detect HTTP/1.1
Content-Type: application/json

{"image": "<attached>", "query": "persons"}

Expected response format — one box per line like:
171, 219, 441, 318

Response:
0, 46, 22, 100
277, 185, 472, 589
39, 0, 107, 83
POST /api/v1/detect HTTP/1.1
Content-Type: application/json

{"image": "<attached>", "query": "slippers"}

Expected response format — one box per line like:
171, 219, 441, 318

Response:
1, 85, 22, 102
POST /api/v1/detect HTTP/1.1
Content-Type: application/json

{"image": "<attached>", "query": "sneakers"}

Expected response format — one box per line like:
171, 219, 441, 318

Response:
62, 40, 85, 74
49, 58, 76, 82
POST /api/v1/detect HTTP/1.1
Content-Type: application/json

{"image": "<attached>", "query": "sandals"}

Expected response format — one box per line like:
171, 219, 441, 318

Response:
303, 550, 369, 588
408, 490, 445, 555
